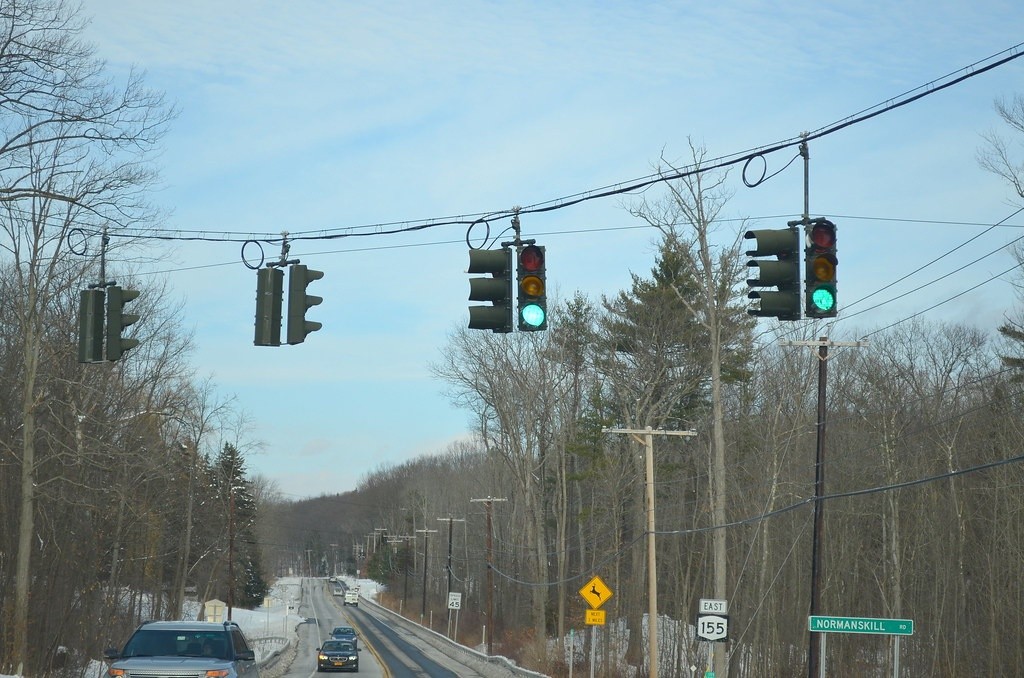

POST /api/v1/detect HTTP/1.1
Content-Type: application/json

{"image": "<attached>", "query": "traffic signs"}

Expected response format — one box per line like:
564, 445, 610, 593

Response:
808, 615, 915, 637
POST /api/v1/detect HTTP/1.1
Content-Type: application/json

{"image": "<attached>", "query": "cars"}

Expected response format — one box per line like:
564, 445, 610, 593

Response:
329, 577, 337, 583
328, 625, 359, 648
333, 587, 343, 597
316, 640, 361, 672
100, 619, 262, 678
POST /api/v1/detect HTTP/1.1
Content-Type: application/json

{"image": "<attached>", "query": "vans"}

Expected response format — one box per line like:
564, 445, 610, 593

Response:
344, 590, 358, 608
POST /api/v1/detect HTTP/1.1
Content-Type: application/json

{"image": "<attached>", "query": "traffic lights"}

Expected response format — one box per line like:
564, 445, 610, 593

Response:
744, 226, 803, 322
515, 244, 548, 334
107, 286, 140, 362
287, 264, 324, 345
466, 246, 514, 335
804, 219, 839, 320
